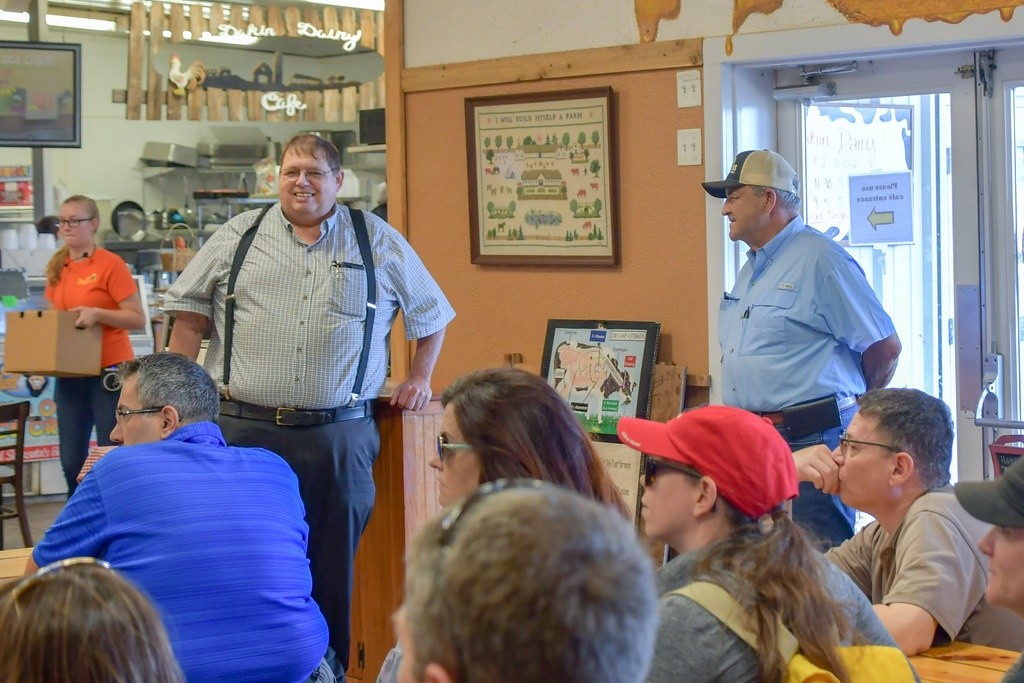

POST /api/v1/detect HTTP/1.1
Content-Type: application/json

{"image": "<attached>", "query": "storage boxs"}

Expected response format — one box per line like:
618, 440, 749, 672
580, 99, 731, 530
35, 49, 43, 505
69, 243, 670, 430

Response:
4, 310, 104, 377
139, 140, 199, 169
358, 107, 385, 145
136, 248, 164, 267
196, 139, 266, 158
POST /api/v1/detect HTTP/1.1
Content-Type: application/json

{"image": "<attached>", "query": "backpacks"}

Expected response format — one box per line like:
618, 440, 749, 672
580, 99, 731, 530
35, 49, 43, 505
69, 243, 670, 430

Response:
663, 580, 915, 683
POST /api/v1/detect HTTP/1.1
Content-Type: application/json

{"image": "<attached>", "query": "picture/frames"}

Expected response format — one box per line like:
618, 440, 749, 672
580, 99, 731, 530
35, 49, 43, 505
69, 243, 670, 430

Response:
463, 81, 625, 269
539, 316, 663, 445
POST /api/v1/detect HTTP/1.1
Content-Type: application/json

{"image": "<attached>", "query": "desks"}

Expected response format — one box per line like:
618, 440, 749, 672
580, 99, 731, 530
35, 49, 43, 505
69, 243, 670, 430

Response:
912, 638, 1024, 683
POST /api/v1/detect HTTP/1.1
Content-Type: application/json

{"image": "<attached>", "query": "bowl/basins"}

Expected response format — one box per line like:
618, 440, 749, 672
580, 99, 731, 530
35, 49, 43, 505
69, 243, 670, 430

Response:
0, 223, 54, 250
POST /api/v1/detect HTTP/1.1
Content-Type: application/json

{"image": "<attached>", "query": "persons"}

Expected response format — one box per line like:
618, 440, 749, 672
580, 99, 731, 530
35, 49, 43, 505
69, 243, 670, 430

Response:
46, 195, 146, 501
704, 150, 902, 553
376, 363, 633, 683
24, 350, 346, 683
392, 477, 658, 683
954, 453, 1024, 683
162, 132, 457, 674
0, 555, 189, 682
617, 406, 922, 683
785, 384, 1024, 658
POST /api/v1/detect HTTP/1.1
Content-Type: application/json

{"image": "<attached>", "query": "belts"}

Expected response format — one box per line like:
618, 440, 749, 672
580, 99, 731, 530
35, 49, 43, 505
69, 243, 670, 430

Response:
218, 400, 374, 426
837, 395, 856, 410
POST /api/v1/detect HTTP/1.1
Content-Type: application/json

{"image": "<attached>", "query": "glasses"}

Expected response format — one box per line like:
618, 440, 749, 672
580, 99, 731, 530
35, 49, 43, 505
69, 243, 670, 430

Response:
115, 407, 181, 422
434, 476, 556, 682
646, 456, 717, 514
438, 434, 513, 462
839, 432, 900, 456
55, 217, 94, 227
279, 168, 336, 179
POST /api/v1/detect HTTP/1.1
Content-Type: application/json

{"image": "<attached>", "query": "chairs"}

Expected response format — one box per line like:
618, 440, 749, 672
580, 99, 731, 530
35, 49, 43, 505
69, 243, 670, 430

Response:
0, 399, 36, 548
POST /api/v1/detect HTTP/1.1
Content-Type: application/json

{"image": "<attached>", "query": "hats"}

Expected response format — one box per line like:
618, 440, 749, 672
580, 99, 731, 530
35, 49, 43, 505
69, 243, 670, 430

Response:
619, 405, 800, 519
702, 149, 798, 198
953, 455, 1024, 527
